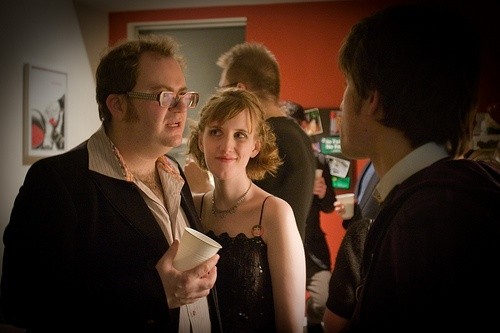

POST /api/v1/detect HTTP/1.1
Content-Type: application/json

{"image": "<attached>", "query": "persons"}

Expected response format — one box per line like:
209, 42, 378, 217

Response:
186, 87, 306, 333
184, 45, 316, 243
279, 100, 337, 333
469, 105, 500, 173
211, 181, 252, 217
307, 159, 383, 333
339, 5, 500, 333
0, 35, 220, 333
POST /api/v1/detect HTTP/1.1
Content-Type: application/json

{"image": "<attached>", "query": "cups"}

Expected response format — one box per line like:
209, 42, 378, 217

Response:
172, 227, 223, 271
334, 193, 355, 220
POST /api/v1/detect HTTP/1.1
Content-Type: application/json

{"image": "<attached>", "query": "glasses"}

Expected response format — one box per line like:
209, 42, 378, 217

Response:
121, 89, 199, 108
215, 83, 237, 91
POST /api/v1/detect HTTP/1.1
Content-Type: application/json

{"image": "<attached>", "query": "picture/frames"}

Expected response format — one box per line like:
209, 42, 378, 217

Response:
22, 63, 69, 166
